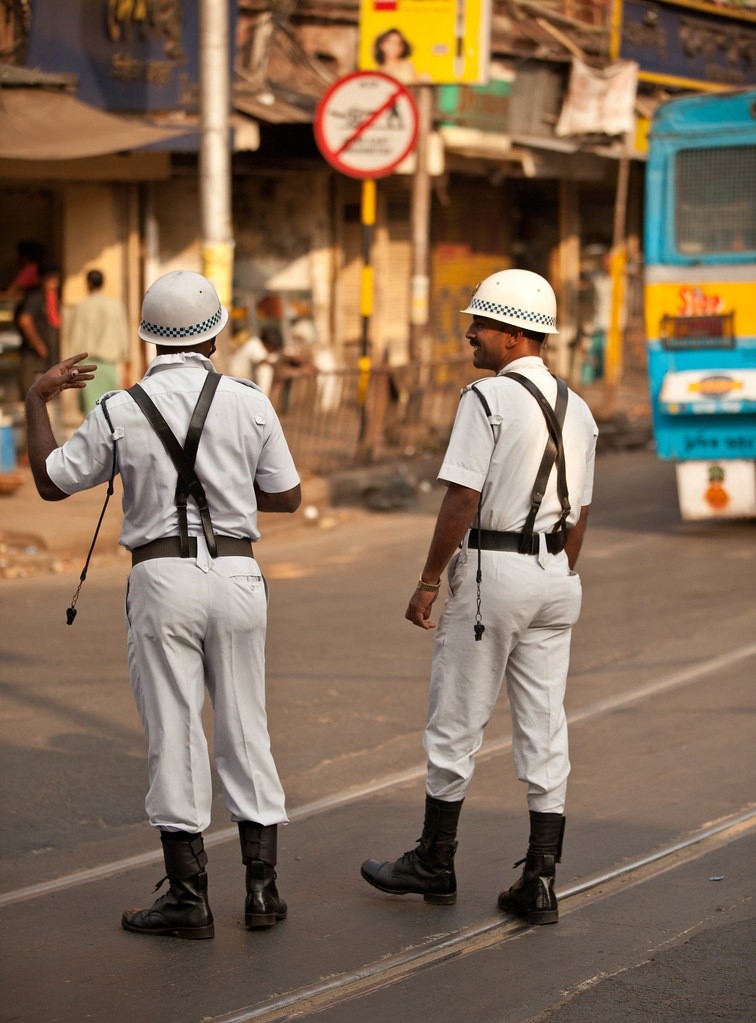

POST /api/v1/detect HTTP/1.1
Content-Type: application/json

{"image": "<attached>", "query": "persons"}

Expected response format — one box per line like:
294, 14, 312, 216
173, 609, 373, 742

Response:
0, 232, 646, 403
374, 27, 414, 83
25, 269, 302, 939
359, 269, 600, 924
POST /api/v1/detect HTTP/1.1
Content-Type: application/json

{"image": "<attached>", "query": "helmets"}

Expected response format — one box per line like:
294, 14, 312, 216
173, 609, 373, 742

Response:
137, 271, 228, 345
459, 269, 561, 334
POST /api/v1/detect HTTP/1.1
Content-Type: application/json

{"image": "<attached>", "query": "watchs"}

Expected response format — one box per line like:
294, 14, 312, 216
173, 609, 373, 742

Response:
417, 573, 442, 590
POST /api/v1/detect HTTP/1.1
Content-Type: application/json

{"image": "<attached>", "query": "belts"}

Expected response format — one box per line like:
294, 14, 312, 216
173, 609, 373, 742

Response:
468, 528, 567, 555
132, 536, 254, 567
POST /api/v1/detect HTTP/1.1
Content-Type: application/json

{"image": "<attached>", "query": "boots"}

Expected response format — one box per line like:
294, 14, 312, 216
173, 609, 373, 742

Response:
121, 831, 215, 938
361, 792, 465, 904
237, 820, 288, 926
497, 810, 566, 924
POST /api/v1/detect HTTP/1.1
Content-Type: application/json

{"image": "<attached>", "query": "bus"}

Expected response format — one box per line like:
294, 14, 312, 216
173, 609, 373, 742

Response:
644, 92, 756, 522
